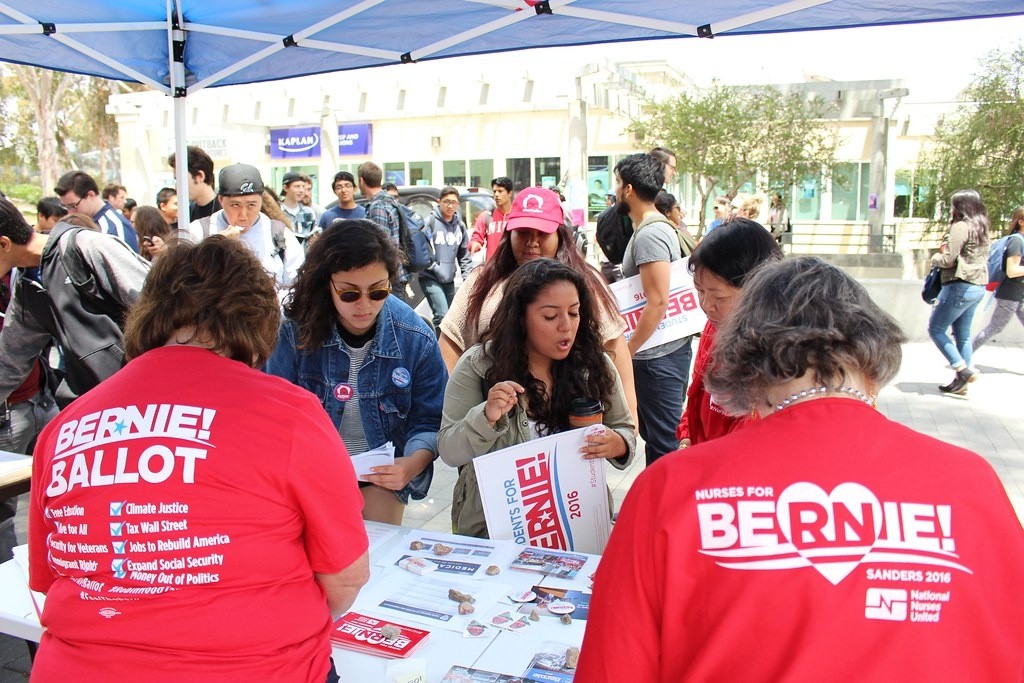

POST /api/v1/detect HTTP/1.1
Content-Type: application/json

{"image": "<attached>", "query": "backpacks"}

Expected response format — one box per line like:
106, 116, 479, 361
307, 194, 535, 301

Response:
593, 202, 635, 266
377, 195, 436, 272
984, 234, 1024, 296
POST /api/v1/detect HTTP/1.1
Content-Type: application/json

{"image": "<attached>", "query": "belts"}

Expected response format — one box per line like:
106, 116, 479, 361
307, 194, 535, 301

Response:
10, 389, 42, 411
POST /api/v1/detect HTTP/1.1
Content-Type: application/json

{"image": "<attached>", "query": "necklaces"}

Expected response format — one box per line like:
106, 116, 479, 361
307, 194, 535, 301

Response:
776, 385, 871, 411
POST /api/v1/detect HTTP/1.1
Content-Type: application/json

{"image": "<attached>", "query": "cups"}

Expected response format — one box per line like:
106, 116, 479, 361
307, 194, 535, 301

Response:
565, 395, 605, 430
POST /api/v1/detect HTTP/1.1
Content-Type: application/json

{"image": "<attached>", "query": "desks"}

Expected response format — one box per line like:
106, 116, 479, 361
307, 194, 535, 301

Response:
0, 520, 604, 683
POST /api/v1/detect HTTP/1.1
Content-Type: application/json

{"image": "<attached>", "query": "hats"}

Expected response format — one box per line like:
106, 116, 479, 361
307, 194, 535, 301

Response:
333, 170, 356, 187
506, 185, 564, 233
606, 189, 617, 197
280, 173, 305, 196
218, 161, 264, 197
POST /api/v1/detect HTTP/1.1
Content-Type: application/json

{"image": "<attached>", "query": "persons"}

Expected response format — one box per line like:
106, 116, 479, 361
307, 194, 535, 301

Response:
707, 192, 789, 242
596, 148, 695, 468
262, 161, 513, 337
0, 146, 304, 523
927, 191, 990, 395
29, 235, 370, 683
972, 206, 1024, 351
439, 257, 637, 539
676, 218, 782, 450
265, 219, 448, 525
440, 187, 638, 436
572, 256, 1024, 683
550, 185, 571, 226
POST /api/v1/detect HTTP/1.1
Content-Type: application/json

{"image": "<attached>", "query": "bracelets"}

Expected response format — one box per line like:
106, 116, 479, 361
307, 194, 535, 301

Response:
678, 444, 689, 448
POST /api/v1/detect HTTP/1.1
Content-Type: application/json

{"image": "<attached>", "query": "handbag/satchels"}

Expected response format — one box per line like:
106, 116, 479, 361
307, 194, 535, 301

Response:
630, 216, 703, 337
922, 267, 943, 305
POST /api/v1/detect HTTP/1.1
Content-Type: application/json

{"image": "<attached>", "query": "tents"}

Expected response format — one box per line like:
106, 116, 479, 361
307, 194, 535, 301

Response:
0, 0, 1024, 241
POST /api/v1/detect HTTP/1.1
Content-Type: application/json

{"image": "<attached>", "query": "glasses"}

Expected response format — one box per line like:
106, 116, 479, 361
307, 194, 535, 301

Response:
329, 275, 392, 303
665, 163, 676, 172
671, 206, 680, 212
57, 192, 86, 213
440, 199, 460, 206
714, 206, 723, 209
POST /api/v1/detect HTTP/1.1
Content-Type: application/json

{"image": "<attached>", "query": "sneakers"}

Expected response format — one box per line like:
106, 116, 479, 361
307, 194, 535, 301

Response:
951, 369, 976, 394
939, 377, 967, 395
